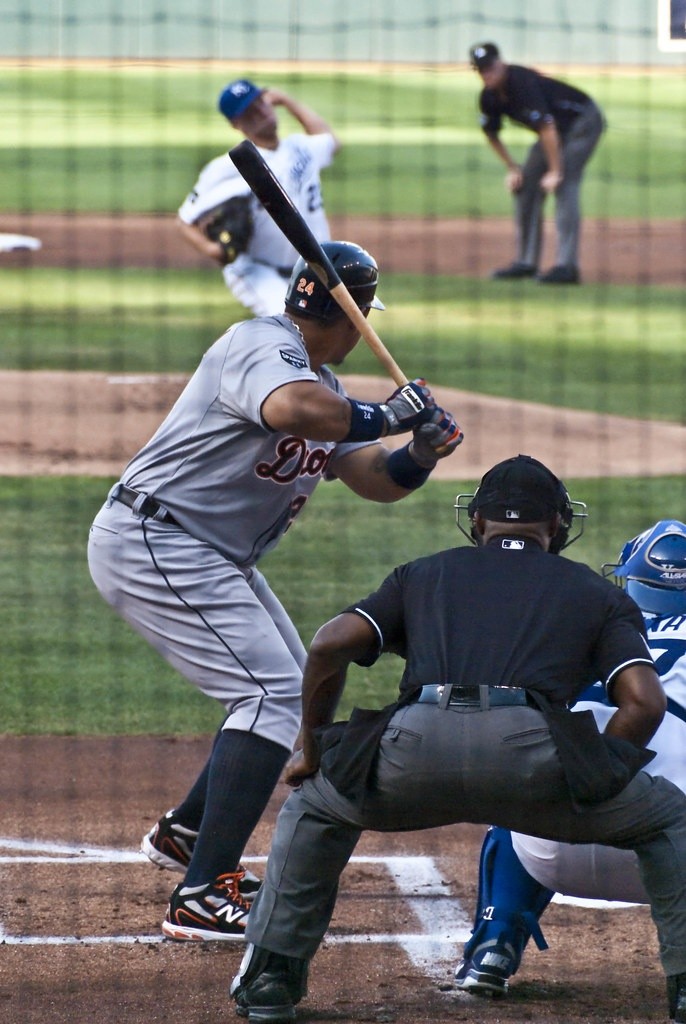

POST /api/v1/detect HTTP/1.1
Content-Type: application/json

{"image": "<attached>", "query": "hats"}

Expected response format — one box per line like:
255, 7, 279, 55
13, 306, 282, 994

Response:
476, 456, 562, 521
219, 79, 265, 121
469, 42, 499, 67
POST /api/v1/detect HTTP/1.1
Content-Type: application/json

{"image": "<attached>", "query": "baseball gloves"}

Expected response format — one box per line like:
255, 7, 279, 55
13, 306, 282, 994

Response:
181, 177, 253, 255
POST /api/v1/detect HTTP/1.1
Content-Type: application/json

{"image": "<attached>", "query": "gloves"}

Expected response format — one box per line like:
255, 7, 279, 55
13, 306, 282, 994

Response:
378, 377, 437, 437
407, 405, 463, 470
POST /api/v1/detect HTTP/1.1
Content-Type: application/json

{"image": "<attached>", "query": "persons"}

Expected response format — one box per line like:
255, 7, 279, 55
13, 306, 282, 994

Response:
454, 519, 686, 999
470, 42, 608, 284
229, 455, 686, 1024
175, 80, 341, 317
87, 241, 464, 941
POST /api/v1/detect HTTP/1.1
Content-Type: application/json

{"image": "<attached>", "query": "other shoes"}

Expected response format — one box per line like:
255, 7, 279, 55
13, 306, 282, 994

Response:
463, 936, 522, 995
493, 265, 535, 278
541, 263, 580, 283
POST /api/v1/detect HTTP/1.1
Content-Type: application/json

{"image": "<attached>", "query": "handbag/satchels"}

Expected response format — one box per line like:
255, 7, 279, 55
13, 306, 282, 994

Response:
527, 689, 657, 808
307, 694, 407, 811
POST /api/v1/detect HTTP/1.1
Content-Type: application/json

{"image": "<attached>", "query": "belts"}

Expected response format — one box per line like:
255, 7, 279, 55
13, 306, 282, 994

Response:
113, 485, 178, 524
412, 685, 536, 706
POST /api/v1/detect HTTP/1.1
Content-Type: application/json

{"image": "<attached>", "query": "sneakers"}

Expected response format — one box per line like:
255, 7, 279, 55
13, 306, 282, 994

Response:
235, 956, 297, 1021
674, 989, 686, 1024
140, 808, 262, 901
160, 873, 251, 942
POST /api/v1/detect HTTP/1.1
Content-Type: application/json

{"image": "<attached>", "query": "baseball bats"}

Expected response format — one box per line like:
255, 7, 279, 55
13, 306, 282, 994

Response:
229, 140, 408, 393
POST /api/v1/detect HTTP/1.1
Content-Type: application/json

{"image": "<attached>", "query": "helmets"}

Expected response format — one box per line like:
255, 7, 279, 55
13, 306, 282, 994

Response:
286, 239, 387, 320
600, 518, 686, 616
